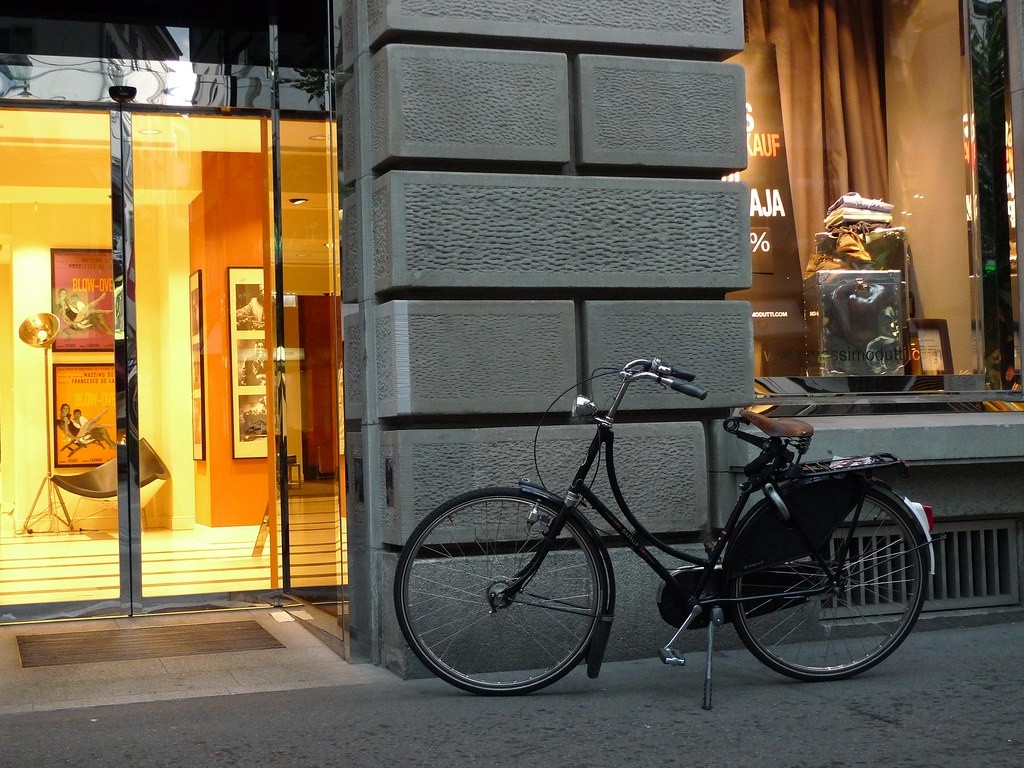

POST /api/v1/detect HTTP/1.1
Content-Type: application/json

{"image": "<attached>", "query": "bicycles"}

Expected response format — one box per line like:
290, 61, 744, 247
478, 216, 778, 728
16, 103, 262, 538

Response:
392, 356, 948, 711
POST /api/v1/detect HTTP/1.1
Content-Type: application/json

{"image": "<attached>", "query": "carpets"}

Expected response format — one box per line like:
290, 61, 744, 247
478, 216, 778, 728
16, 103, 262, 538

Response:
16, 621, 287, 669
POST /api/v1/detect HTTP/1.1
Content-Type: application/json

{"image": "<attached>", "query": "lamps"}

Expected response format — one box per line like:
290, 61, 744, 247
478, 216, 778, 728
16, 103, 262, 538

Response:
19, 312, 76, 533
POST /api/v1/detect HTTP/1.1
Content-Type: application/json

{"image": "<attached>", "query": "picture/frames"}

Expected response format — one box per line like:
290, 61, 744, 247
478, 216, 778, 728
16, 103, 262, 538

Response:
50, 247, 115, 352
227, 266, 267, 459
907, 318, 955, 376
53, 363, 121, 466
188, 268, 206, 462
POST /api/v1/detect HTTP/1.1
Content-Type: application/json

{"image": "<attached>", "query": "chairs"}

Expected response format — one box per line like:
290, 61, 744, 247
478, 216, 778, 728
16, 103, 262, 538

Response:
50, 436, 172, 535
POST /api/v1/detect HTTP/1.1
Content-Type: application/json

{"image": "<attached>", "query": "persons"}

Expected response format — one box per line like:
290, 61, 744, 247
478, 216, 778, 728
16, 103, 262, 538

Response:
58, 288, 114, 335
60, 404, 117, 449
236, 285, 266, 330
242, 340, 266, 385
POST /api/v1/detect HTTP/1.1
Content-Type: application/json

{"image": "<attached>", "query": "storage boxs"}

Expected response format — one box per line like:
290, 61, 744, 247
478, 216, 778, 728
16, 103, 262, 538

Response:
803, 270, 903, 378
